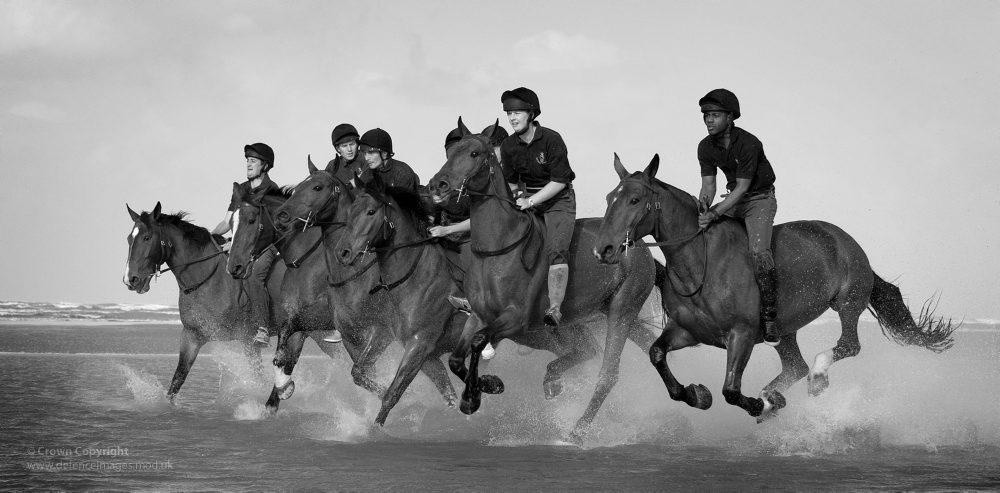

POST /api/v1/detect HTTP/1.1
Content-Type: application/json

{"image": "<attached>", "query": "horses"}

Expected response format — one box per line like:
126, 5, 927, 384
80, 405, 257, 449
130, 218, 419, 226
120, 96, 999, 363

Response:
225, 182, 336, 413
123, 203, 354, 400
336, 172, 597, 429
273, 153, 396, 401
591, 152, 965, 424
426, 115, 666, 449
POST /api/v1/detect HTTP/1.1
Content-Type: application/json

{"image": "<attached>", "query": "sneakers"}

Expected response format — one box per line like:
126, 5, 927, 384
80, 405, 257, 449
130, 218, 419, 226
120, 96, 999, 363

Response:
480, 340, 497, 361
323, 329, 343, 344
544, 306, 564, 334
761, 312, 784, 347
447, 294, 472, 316
251, 327, 272, 348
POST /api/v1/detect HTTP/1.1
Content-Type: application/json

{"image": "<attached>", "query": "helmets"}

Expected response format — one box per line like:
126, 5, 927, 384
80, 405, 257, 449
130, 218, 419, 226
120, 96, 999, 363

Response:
499, 86, 543, 113
331, 124, 360, 145
355, 128, 394, 155
243, 142, 274, 166
480, 123, 508, 148
444, 127, 472, 149
698, 88, 740, 118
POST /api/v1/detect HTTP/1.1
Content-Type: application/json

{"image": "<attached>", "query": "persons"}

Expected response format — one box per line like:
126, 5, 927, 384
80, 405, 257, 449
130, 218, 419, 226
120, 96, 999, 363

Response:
212, 143, 278, 345
421, 127, 474, 239
325, 123, 371, 343
448, 87, 577, 326
427, 125, 509, 360
697, 88, 780, 347
342, 129, 422, 198
